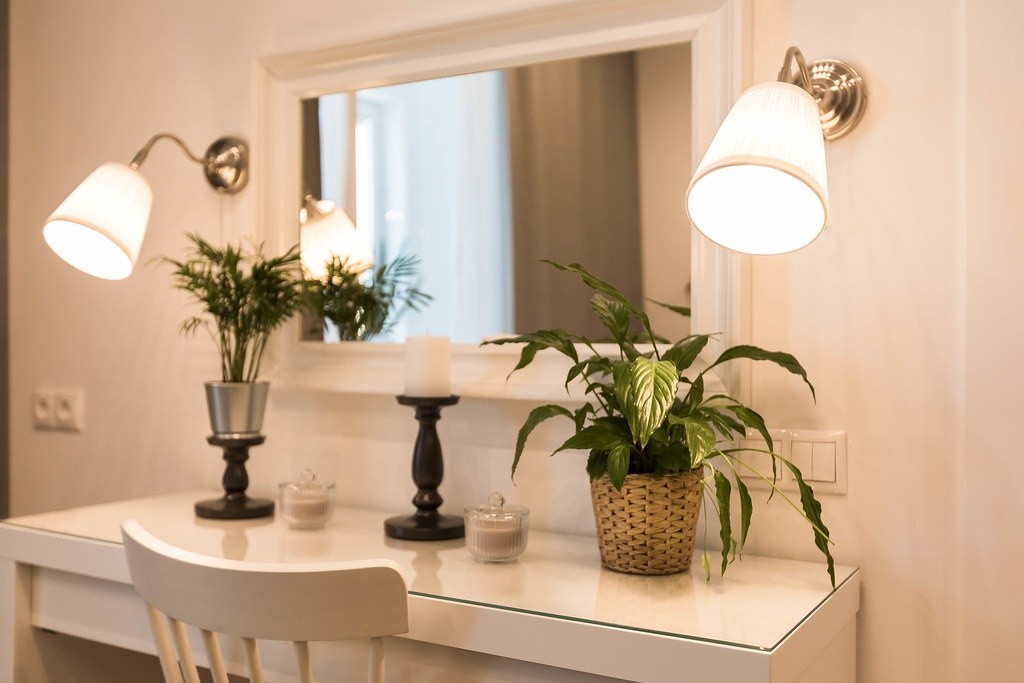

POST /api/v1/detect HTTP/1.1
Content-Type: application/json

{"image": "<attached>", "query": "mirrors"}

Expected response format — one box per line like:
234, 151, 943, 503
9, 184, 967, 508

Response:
300, 42, 693, 344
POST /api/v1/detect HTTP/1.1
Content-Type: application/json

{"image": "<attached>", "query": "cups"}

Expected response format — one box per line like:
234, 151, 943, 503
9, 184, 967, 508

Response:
463, 491, 531, 567
277, 471, 335, 531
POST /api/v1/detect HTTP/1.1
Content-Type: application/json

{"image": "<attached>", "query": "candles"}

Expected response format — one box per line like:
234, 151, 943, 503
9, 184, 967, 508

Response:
402, 330, 455, 397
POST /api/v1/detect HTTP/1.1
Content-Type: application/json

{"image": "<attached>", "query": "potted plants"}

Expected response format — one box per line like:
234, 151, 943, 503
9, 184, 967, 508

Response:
154, 232, 301, 520
478, 260, 838, 592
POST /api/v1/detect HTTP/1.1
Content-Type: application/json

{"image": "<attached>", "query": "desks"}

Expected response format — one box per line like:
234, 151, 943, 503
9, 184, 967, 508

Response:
0, 489, 860, 683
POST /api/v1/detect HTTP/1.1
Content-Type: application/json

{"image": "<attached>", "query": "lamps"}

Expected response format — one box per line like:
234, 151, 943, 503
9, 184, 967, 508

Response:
686, 46, 867, 256
300, 197, 374, 293
43, 133, 249, 281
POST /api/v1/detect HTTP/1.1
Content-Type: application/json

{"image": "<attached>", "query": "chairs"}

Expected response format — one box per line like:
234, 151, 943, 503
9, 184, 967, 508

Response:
122, 521, 409, 682
